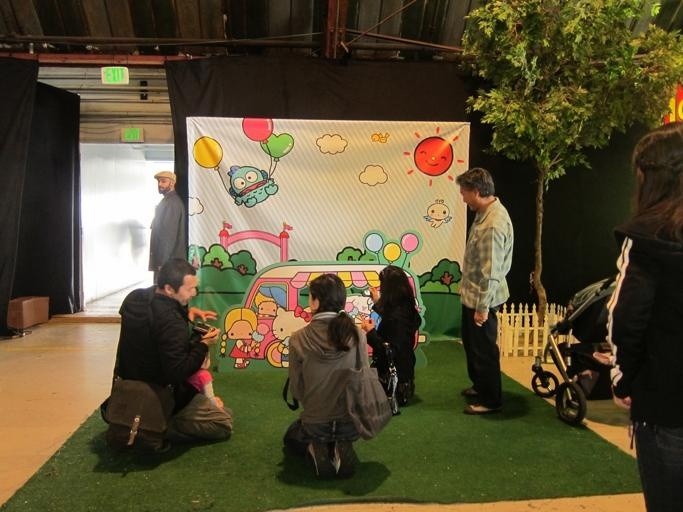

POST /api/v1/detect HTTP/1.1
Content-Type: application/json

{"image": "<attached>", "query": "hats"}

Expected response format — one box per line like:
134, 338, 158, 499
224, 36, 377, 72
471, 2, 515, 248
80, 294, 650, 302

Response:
154, 171, 176, 181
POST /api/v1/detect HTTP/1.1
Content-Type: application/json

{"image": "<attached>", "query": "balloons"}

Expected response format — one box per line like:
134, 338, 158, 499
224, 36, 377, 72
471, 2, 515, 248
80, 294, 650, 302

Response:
242, 117, 274, 144
260, 133, 294, 161
364, 231, 384, 254
193, 136, 223, 170
382, 243, 401, 263
400, 233, 419, 253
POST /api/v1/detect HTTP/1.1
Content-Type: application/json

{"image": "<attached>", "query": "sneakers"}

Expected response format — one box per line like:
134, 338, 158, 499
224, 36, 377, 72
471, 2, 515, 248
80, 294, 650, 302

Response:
461, 386, 503, 415
309, 437, 357, 478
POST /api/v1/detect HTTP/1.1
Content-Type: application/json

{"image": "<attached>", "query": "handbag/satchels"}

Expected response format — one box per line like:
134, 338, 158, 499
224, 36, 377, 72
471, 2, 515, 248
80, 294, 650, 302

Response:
106, 379, 174, 451
347, 366, 392, 441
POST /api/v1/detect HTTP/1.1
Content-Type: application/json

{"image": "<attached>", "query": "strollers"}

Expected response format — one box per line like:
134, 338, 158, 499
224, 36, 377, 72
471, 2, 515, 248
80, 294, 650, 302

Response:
528, 272, 612, 426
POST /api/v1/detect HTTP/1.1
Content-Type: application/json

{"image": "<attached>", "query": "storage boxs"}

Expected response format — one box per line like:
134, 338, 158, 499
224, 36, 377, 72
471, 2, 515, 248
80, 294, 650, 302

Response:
7, 296, 50, 330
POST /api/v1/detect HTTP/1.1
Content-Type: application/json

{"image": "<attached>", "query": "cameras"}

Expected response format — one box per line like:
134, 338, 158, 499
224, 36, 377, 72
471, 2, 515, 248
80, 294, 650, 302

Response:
193, 320, 217, 338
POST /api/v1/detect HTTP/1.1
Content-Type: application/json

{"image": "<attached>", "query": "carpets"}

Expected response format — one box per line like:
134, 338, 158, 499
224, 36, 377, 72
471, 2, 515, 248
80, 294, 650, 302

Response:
0, 339, 643, 512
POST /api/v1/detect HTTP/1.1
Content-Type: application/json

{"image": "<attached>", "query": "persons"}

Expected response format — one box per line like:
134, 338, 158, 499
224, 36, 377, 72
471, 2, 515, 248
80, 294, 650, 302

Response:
185, 349, 224, 411
101, 257, 233, 467
283, 274, 370, 480
369, 265, 422, 408
148, 172, 189, 286
456, 167, 515, 415
606, 122, 683, 512
